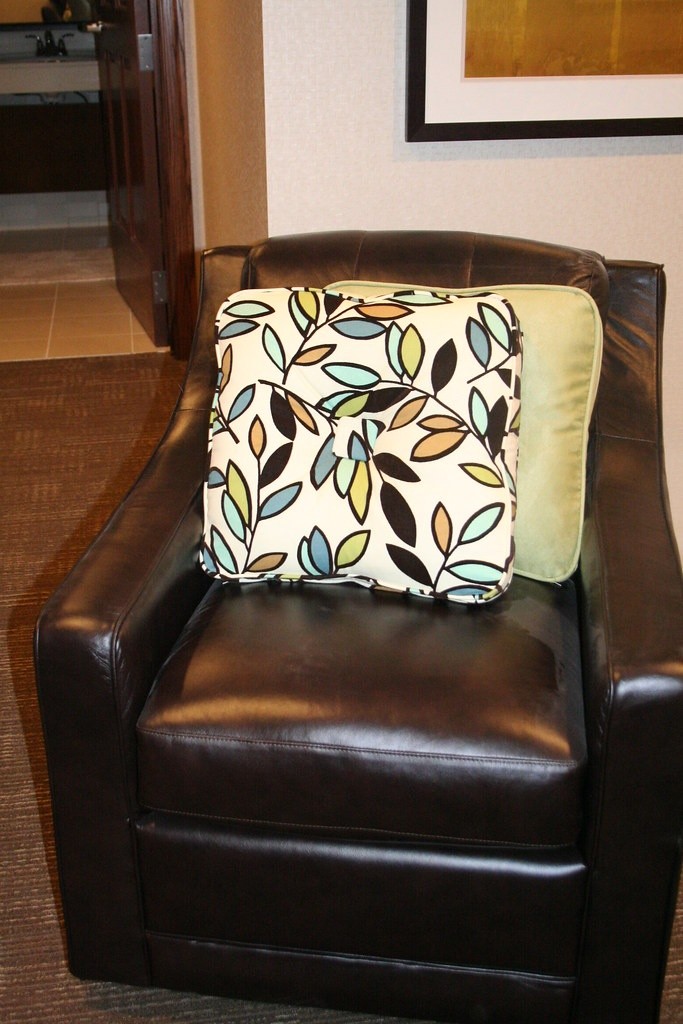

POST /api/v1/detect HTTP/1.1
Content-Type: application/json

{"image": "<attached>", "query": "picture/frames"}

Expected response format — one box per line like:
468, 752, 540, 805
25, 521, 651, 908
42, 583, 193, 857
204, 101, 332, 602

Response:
403, 0, 683, 143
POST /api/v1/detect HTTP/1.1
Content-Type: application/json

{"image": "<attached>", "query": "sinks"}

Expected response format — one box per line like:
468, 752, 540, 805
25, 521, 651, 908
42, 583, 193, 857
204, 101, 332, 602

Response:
0, 60, 101, 95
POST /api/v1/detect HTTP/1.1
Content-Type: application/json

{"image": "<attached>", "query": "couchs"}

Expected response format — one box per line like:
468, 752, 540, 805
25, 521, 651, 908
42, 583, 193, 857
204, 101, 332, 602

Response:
30, 229, 683, 1024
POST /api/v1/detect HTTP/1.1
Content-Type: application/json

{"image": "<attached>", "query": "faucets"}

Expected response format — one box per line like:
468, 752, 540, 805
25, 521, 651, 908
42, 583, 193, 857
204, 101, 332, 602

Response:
44, 30, 56, 48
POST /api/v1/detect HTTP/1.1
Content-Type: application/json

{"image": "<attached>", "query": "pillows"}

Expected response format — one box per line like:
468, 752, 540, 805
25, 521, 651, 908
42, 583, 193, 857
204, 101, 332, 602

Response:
325, 280, 604, 583
199, 287, 526, 606
